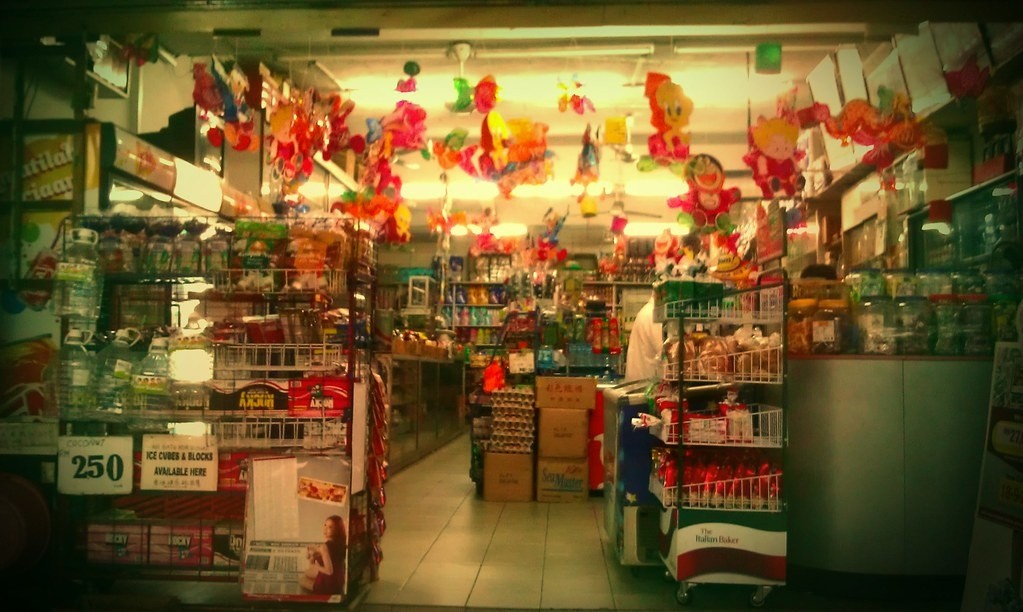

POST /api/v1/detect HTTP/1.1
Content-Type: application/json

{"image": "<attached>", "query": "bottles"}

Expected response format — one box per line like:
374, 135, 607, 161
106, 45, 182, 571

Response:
789, 267, 991, 357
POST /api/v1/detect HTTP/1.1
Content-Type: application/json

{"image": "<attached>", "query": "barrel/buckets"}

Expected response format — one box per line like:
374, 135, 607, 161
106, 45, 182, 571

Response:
46, 329, 93, 421
128, 338, 177, 432
50, 229, 105, 318
86, 328, 142, 423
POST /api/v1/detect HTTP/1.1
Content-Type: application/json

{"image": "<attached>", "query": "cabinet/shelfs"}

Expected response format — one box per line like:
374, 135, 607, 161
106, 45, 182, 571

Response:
46, 167, 1023, 612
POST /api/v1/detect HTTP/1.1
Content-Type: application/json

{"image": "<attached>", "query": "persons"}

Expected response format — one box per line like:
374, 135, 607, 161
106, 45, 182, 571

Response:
625, 285, 667, 382
301, 515, 345, 594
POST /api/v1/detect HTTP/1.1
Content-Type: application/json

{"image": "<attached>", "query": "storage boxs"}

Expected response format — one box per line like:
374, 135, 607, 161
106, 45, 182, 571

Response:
534, 374, 596, 410
482, 450, 536, 502
537, 457, 589, 504
538, 406, 589, 460
